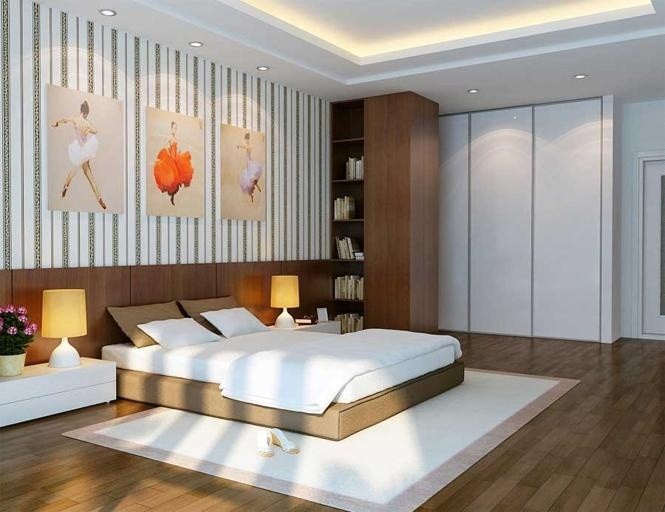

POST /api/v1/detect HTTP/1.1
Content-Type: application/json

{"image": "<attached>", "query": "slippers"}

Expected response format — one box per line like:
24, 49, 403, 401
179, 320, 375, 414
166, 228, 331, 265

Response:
269, 426, 300, 455
257, 429, 273, 457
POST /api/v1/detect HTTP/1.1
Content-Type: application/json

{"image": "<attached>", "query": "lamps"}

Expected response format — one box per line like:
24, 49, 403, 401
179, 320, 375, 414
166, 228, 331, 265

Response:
269, 275, 300, 329
41, 288, 90, 371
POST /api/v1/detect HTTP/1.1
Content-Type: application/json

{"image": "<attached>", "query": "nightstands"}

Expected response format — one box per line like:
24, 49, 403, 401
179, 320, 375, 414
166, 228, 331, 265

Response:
266, 319, 340, 334
0, 356, 122, 432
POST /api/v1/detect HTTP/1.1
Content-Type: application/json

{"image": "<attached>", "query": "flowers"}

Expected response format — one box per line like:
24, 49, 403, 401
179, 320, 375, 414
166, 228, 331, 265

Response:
0, 305, 34, 350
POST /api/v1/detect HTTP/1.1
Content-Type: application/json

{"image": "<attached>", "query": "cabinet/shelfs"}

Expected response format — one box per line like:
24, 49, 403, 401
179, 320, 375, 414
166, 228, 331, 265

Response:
328, 90, 439, 341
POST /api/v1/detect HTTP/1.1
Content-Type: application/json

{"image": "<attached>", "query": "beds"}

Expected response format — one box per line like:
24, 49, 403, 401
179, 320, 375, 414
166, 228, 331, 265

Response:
100, 294, 465, 428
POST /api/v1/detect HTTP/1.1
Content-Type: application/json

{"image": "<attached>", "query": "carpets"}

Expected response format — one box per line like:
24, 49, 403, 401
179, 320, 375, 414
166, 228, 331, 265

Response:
56, 368, 582, 511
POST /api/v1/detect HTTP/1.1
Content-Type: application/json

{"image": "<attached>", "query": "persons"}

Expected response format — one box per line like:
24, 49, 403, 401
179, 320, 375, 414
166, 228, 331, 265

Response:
235, 132, 264, 202
153, 122, 194, 206
48, 99, 107, 209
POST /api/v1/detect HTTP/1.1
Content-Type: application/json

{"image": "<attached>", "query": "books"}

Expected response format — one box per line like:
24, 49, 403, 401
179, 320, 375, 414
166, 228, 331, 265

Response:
295, 315, 316, 325
329, 155, 363, 333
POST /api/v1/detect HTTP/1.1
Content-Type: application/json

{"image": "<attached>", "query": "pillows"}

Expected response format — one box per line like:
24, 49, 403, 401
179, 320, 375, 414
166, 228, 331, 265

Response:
180, 299, 249, 335
102, 303, 183, 343
126, 316, 222, 344
193, 305, 275, 335
